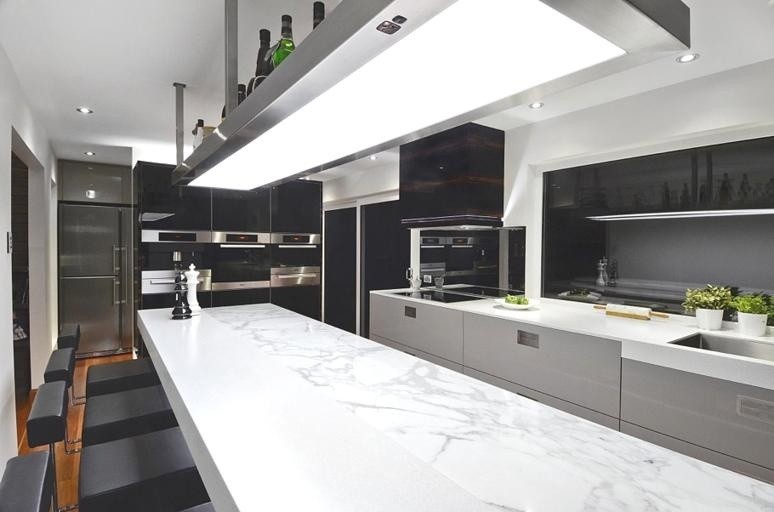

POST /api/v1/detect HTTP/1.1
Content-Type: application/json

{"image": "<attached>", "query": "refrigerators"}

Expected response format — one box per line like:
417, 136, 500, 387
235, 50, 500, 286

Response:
57, 157, 134, 360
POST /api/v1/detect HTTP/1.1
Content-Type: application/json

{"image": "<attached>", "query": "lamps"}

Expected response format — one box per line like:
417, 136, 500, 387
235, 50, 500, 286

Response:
76, 108, 92, 114
676, 53, 699, 64
528, 101, 544, 109
368, 156, 377, 161
85, 152, 95, 156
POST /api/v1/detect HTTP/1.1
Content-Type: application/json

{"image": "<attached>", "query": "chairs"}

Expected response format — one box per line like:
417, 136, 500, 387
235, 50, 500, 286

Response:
28, 381, 212, 512
2, 450, 216, 512
57, 323, 160, 394
44, 348, 179, 446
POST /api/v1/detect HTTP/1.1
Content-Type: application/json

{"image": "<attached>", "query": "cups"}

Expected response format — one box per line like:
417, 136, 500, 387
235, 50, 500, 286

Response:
435, 278, 444, 291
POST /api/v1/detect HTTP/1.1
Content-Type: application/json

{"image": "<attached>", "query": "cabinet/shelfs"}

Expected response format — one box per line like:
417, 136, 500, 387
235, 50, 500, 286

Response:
369, 284, 774, 485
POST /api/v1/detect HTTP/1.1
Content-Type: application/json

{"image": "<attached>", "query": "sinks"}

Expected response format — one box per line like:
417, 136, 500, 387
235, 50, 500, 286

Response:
666, 329, 773, 364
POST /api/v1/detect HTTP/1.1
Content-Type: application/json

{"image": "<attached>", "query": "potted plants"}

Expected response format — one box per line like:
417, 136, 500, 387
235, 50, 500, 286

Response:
682, 285, 738, 331
731, 293, 773, 336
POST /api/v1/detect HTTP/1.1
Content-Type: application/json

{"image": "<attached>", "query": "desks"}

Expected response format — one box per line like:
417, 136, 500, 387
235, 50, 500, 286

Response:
137, 302, 774, 510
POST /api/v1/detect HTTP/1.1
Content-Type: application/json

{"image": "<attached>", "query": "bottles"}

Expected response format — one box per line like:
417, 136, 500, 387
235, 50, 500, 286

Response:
238, 84, 246, 106
313, 1, 325, 30
272, 15, 296, 69
193, 119, 204, 149
255, 28, 273, 91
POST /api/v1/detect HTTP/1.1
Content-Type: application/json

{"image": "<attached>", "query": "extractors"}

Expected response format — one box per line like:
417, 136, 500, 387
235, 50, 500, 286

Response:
397, 121, 504, 233
